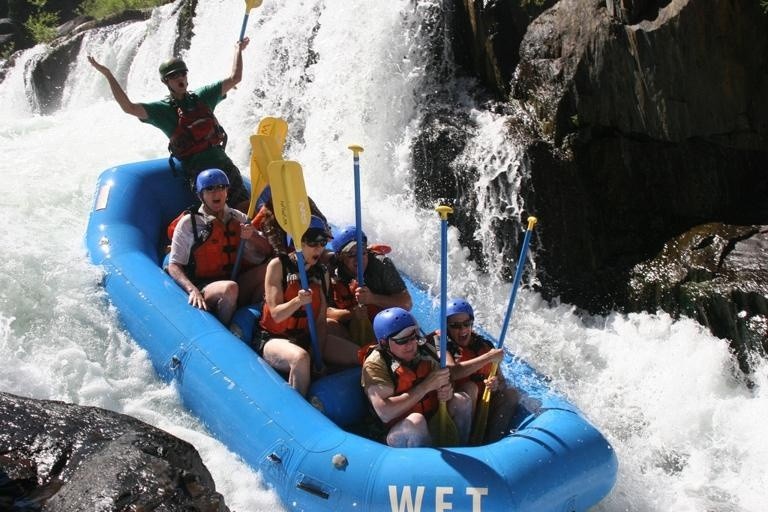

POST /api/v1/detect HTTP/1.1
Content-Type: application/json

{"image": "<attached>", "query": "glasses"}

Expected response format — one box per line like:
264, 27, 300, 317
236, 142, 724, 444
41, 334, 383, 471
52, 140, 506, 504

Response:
348, 248, 365, 258
166, 72, 187, 80
306, 239, 328, 247
388, 329, 418, 344
446, 317, 473, 328
205, 185, 226, 192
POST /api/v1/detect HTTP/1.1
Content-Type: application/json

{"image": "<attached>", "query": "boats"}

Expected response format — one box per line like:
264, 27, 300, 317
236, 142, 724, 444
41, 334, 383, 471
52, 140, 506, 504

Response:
82, 150, 619, 511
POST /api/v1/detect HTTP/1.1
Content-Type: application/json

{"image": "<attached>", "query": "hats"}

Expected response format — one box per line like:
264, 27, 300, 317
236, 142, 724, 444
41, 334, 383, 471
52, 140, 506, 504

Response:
196, 169, 230, 192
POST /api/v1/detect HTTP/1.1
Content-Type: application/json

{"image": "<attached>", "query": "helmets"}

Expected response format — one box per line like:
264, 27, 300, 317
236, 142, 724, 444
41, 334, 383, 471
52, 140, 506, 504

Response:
446, 298, 474, 321
159, 58, 189, 78
331, 224, 367, 255
307, 215, 326, 232
373, 308, 416, 346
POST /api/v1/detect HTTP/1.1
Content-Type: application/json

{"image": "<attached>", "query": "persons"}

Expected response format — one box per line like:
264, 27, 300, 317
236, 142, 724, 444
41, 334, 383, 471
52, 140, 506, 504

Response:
418, 299, 518, 446
171, 168, 269, 328
361, 307, 473, 449
330, 224, 413, 346
86, 37, 250, 215
250, 215, 362, 397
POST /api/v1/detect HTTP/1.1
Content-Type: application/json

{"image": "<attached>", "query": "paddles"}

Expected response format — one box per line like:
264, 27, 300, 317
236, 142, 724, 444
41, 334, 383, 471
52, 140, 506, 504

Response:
251, 135, 283, 185
231, 116, 288, 279
267, 161, 323, 370
430, 206, 459, 447
238, 1, 262, 42
348, 146, 373, 344
471, 216, 538, 443
